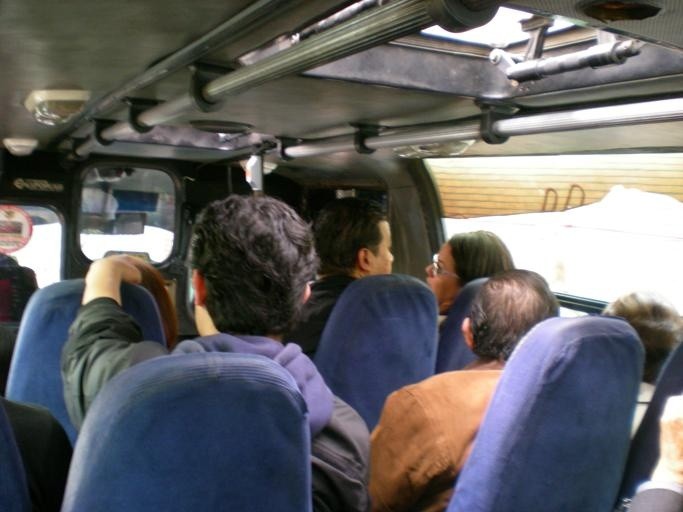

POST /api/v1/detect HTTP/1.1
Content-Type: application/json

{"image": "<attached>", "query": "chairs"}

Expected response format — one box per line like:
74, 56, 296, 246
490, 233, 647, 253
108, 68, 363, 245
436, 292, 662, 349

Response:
439, 278, 491, 369
5, 281, 165, 449
446, 314, 644, 512
622, 340, 682, 511
60, 353, 312, 512
313, 273, 439, 434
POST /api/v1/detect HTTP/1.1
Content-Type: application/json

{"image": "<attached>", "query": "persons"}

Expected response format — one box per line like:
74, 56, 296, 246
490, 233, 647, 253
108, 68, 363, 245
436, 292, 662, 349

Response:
293, 197, 396, 346
367, 265, 561, 510
64, 192, 368, 512
625, 391, 682, 511
419, 233, 514, 313
600, 289, 683, 441
105, 251, 179, 352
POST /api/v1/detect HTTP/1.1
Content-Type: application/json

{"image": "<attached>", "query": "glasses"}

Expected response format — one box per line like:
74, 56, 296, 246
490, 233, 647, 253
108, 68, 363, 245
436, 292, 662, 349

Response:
432, 254, 457, 280
